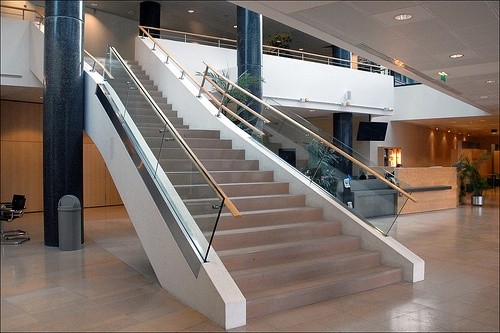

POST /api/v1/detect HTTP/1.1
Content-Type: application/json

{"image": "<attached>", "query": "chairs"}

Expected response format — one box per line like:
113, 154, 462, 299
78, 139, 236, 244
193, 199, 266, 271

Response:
0, 194, 30, 246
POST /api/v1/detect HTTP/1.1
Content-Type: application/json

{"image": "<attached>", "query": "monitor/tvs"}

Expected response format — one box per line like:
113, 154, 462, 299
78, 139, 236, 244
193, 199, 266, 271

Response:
356, 121, 388, 141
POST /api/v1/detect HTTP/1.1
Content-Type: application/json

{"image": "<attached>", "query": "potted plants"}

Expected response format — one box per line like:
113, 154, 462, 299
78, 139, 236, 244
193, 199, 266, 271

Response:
456, 154, 493, 206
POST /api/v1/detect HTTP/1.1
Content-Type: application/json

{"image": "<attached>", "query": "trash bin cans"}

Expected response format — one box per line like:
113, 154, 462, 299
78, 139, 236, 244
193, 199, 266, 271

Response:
57, 195, 82, 251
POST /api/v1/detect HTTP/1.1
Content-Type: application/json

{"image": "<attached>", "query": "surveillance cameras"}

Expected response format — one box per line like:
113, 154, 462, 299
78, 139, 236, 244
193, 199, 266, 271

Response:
344, 90, 351, 100
343, 101, 350, 108
